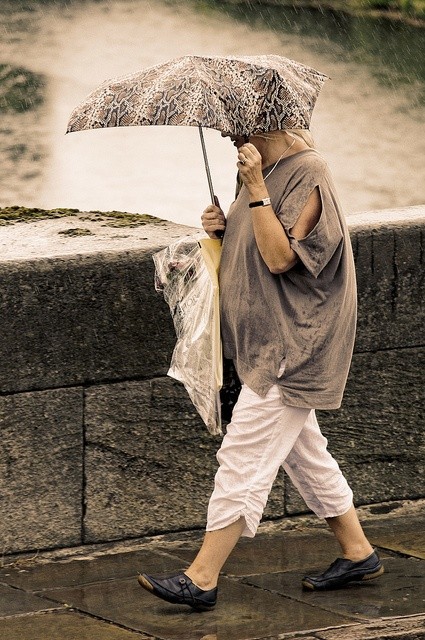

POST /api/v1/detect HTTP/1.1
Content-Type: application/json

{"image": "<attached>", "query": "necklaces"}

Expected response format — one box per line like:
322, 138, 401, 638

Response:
262, 132, 297, 182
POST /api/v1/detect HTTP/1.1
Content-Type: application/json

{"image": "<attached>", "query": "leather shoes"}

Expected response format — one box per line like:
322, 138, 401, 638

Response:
137, 572, 217, 610
302, 548, 384, 589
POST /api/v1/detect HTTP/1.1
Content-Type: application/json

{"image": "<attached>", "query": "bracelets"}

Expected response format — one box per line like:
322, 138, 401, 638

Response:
247, 198, 273, 209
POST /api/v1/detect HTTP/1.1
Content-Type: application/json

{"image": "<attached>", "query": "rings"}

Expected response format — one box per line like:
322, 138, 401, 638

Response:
240, 158, 248, 167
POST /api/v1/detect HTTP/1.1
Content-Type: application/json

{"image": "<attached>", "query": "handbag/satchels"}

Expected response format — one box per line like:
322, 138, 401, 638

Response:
152, 238, 241, 436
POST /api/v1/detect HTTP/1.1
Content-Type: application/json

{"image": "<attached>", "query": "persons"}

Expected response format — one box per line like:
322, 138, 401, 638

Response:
139, 124, 384, 612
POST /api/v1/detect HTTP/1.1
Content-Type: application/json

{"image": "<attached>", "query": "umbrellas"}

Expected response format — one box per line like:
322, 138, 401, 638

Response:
63, 54, 334, 240
